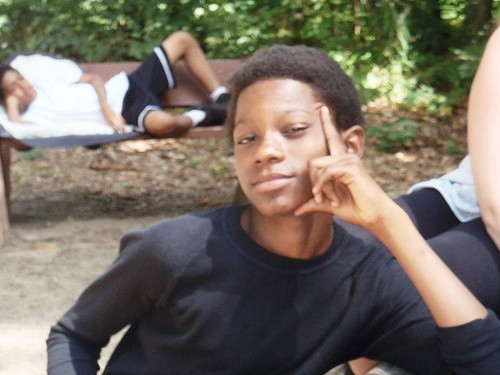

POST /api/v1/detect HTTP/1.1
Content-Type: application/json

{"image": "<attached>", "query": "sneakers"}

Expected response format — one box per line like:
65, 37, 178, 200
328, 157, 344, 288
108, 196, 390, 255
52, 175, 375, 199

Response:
187, 92, 234, 128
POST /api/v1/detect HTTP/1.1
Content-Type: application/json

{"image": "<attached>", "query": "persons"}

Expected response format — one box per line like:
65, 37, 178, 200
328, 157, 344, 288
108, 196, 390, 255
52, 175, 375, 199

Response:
0, 30, 233, 138
46, 44, 500, 375
321, 25, 500, 375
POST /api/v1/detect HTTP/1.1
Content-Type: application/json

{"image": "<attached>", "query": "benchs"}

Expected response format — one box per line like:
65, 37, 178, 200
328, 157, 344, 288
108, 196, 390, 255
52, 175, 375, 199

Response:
0, 57, 249, 230
